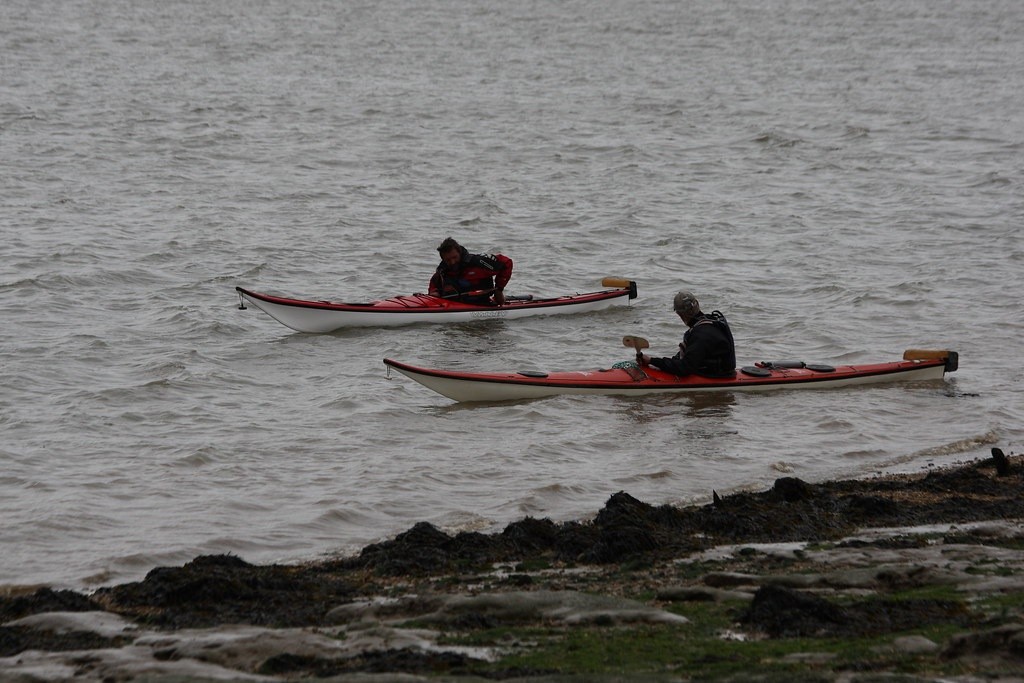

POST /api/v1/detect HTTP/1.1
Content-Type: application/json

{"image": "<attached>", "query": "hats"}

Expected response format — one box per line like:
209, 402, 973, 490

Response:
674, 292, 698, 310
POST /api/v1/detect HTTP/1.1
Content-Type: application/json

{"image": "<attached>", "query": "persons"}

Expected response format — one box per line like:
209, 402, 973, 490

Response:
428, 237, 513, 306
636, 291, 737, 378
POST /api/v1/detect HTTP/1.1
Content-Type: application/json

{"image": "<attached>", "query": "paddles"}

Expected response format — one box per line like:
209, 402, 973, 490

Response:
622, 336, 650, 363
438, 287, 500, 299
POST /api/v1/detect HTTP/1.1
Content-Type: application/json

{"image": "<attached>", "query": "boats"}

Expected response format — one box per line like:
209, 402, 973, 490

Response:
233, 274, 638, 337
380, 350, 954, 406
